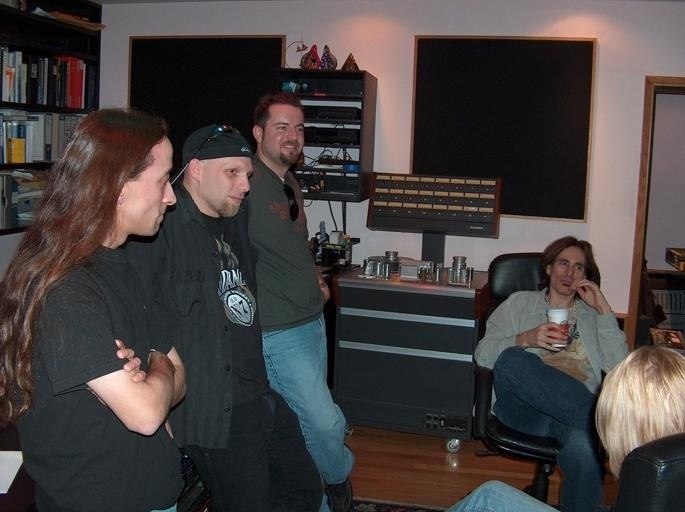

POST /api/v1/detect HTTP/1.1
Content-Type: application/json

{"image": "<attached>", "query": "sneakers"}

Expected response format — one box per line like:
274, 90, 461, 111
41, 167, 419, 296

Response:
327, 480, 353, 511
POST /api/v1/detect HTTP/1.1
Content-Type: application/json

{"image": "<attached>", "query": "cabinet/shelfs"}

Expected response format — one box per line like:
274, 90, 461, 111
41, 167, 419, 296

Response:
273, 67, 377, 203
0, 0, 104, 236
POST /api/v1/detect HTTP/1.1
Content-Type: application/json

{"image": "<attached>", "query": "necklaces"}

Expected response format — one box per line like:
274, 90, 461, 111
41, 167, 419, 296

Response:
544, 293, 576, 322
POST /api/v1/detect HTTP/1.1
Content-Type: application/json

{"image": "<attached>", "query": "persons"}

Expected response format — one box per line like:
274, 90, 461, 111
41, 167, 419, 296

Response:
444, 343, 685, 512
124, 122, 323, 511
1, 108, 186, 512
245, 91, 359, 512
473, 235, 631, 512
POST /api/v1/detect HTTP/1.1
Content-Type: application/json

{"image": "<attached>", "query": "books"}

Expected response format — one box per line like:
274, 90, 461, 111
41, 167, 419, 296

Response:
648, 326, 685, 356
0, 1, 109, 234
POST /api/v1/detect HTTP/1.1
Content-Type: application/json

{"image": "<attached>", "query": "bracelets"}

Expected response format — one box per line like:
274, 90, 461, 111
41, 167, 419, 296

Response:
319, 282, 328, 288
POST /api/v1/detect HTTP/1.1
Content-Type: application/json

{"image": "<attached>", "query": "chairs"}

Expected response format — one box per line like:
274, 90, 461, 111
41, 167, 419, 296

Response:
472, 252, 608, 504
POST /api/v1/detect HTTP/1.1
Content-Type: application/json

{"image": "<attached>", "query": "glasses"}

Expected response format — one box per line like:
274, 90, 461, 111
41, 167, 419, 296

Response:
197, 126, 232, 155
284, 184, 299, 222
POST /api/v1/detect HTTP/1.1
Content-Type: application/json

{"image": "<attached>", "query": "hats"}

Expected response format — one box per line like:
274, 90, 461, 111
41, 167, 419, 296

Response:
183, 126, 253, 164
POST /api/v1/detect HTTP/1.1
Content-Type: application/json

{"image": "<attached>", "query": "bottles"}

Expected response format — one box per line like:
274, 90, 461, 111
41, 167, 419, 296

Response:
384, 251, 399, 274
446, 256, 474, 283
418, 262, 443, 284
319, 221, 326, 238
362, 258, 392, 281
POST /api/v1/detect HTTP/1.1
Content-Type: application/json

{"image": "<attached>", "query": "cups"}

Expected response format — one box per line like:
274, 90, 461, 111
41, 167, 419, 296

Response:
547, 308, 569, 347
